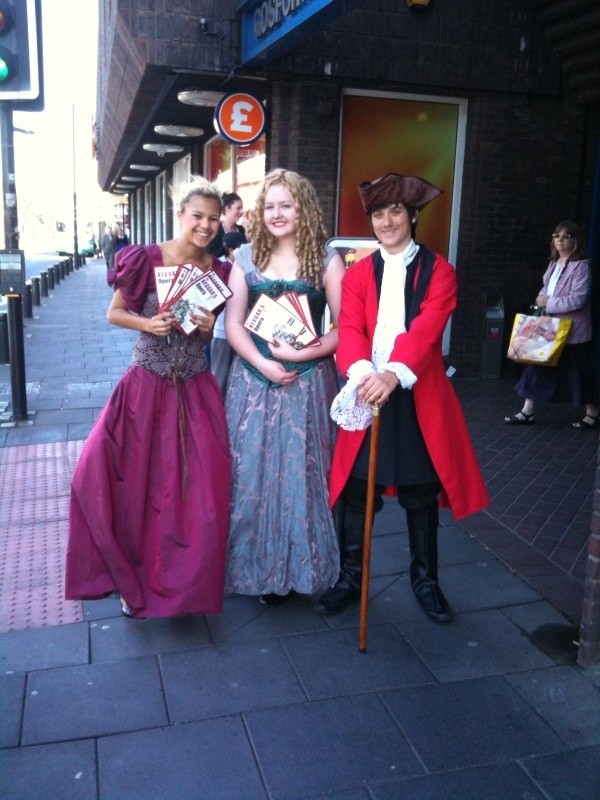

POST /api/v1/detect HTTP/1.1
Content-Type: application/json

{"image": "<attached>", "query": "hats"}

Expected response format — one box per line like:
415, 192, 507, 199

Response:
357, 171, 443, 216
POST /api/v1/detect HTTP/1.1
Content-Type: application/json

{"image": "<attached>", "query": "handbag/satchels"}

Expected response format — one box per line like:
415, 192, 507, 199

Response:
506, 307, 573, 368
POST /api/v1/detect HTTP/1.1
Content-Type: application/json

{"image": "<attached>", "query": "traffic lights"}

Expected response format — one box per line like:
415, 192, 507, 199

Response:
0, 0, 40, 101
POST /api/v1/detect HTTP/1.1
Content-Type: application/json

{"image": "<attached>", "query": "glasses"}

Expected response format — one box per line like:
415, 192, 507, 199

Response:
552, 233, 575, 239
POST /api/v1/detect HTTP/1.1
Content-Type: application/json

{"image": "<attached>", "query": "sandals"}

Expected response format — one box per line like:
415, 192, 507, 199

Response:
504, 410, 535, 425
571, 414, 600, 429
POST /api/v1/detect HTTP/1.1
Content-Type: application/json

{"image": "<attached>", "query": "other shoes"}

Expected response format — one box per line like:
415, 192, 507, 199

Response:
259, 590, 293, 605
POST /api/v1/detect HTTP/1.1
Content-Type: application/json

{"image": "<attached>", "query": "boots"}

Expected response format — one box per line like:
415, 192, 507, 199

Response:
406, 506, 455, 623
312, 502, 366, 613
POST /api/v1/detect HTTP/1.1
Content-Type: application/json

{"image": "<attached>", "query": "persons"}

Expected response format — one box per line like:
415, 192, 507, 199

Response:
64, 177, 232, 616
503, 215, 600, 430
221, 168, 346, 606
206, 192, 247, 391
313, 172, 490, 621
102, 226, 129, 272
